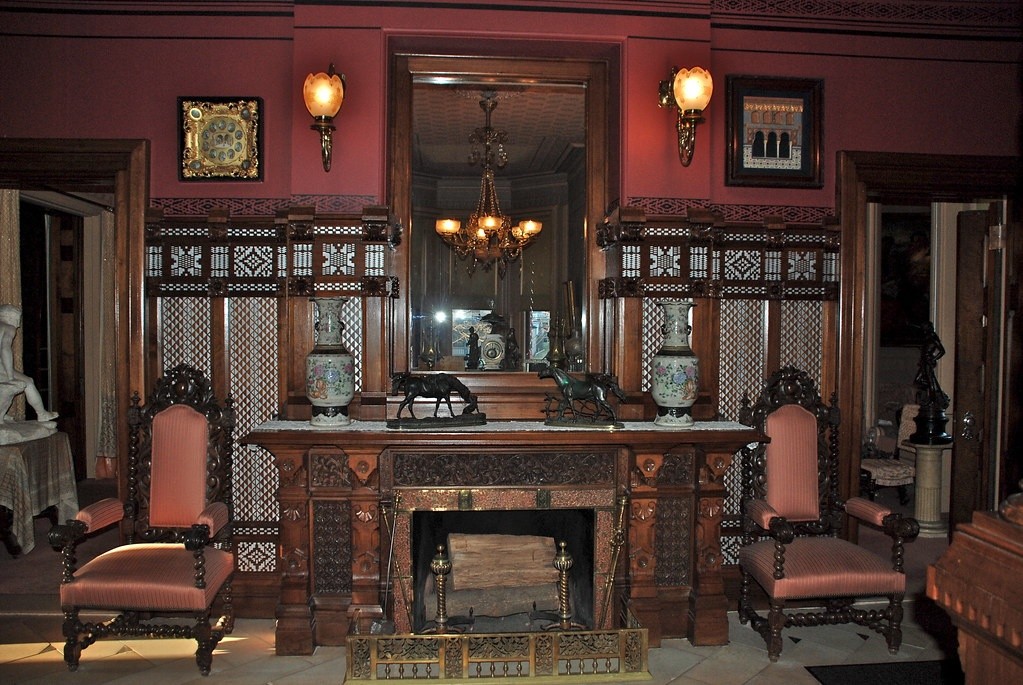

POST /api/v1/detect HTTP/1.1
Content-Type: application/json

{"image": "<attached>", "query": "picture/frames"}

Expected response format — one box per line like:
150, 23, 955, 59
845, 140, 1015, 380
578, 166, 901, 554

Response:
177, 95, 265, 182
724, 74, 825, 189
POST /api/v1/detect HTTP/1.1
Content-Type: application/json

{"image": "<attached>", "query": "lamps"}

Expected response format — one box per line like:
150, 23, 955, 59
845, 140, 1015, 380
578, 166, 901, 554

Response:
303, 63, 346, 172
435, 92, 543, 279
658, 66, 713, 168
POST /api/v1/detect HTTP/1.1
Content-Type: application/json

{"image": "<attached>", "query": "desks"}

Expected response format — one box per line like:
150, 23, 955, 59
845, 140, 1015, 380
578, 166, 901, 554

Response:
237, 407, 772, 655
0, 431, 70, 558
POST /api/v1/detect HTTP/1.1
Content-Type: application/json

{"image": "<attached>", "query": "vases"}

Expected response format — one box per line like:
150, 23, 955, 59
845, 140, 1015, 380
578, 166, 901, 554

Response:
652, 301, 699, 427
305, 296, 355, 428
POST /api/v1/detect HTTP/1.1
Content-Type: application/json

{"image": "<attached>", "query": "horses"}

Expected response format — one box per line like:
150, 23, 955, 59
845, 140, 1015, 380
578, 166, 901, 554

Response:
389, 372, 479, 419
537, 365, 628, 424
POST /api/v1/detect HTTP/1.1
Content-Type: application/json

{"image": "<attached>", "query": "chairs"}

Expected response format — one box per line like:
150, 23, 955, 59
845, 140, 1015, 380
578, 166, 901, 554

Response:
861, 404, 921, 506
48, 363, 236, 676
739, 365, 919, 663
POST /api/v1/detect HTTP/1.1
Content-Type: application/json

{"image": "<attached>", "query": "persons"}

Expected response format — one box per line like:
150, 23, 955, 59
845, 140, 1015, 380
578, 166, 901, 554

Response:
465, 326, 479, 361
913, 321, 945, 398
507, 328, 519, 356
0, 304, 60, 422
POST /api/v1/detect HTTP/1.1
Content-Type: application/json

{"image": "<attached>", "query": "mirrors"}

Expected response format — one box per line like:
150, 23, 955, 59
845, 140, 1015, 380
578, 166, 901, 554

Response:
386, 47, 612, 394
